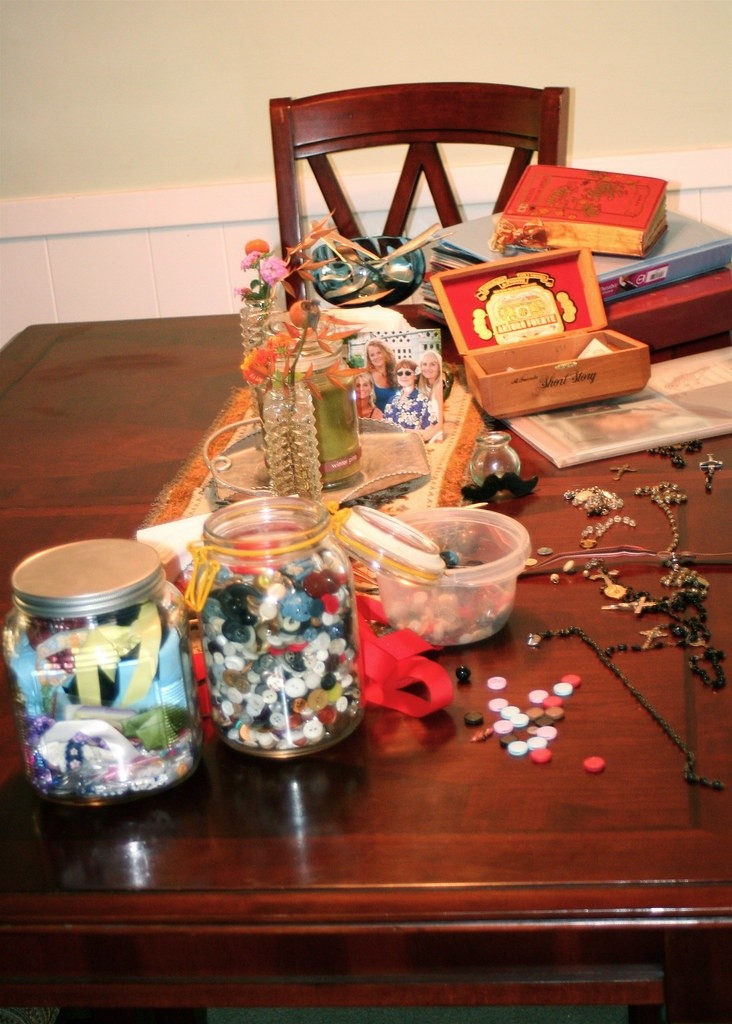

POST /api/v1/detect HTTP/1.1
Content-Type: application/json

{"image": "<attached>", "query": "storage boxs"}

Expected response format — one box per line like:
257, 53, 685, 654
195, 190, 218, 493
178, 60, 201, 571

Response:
425, 244, 652, 421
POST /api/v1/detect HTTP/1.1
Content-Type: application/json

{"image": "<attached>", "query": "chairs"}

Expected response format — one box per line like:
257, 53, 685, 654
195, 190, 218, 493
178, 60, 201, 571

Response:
268, 81, 570, 312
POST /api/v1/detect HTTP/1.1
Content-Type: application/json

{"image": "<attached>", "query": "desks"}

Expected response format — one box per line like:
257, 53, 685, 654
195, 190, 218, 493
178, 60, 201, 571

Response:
1, 303, 732, 1024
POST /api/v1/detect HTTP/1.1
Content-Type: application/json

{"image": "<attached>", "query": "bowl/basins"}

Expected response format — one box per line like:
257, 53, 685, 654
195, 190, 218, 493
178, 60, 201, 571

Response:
380, 504, 532, 645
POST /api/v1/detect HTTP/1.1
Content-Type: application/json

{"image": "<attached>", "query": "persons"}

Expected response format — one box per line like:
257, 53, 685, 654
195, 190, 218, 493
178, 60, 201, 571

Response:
383, 358, 438, 429
366, 339, 401, 413
414, 350, 442, 400
354, 373, 383, 419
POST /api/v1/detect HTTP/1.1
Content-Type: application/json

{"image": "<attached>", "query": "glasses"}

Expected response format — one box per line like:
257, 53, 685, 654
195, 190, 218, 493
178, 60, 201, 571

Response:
397, 370, 413, 376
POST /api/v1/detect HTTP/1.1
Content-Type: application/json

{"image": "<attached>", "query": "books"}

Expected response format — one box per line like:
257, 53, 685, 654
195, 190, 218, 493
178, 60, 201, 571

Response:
420, 166, 732, 352
